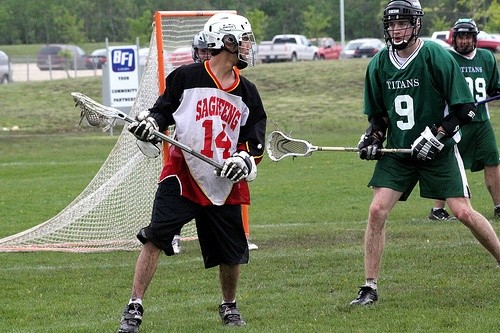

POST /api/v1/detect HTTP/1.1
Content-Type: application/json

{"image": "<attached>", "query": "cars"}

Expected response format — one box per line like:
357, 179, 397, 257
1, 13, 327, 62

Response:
37, 44, 88, 71
84, 48, 107, 70
0, 50, 11, 86
342, 38, 385, 59
431, 30, 500, 53
307, 37, 342, 60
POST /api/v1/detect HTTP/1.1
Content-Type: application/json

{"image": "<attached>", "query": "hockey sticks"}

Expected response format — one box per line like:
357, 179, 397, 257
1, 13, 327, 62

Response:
266, 130, 412, 162
71, 92, 223, 171
474, 95, 500, 107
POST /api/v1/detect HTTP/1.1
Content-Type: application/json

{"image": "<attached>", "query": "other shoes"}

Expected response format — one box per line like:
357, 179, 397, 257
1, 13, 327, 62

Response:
171, 240, 179, 254
246, 241, 258, 250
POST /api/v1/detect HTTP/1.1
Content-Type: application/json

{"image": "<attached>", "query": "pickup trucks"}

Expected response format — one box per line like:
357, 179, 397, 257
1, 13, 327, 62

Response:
257, 34, 321, 64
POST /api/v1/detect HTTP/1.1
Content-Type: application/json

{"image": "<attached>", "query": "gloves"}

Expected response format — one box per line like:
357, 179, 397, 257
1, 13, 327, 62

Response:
410, 123, 449, 161
213, 150, 257, 184
357, 126, 385, 160
128, 111, 159, 142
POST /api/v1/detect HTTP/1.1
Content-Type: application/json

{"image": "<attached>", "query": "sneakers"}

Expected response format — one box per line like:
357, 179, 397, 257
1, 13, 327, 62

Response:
118, 303, 144, 333
495, 206, 500, 216
219, 301, 247, 328
428, 208, 458, 222
349, 286, 377, 307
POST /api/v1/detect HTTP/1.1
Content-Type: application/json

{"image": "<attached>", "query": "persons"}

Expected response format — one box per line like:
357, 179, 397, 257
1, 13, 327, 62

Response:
349, 0, 500, 306
117, 11, 268, 333
428, 18, 500, 222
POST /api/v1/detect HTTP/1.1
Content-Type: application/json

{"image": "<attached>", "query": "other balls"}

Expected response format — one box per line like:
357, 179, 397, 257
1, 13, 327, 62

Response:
87, 114, 100, 126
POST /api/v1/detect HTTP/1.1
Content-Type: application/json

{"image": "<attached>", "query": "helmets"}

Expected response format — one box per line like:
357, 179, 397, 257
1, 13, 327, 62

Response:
204, 12, 257, 70
383, 0, 424, 51
451, 18, 479, 54
192, 32, 211, 62
